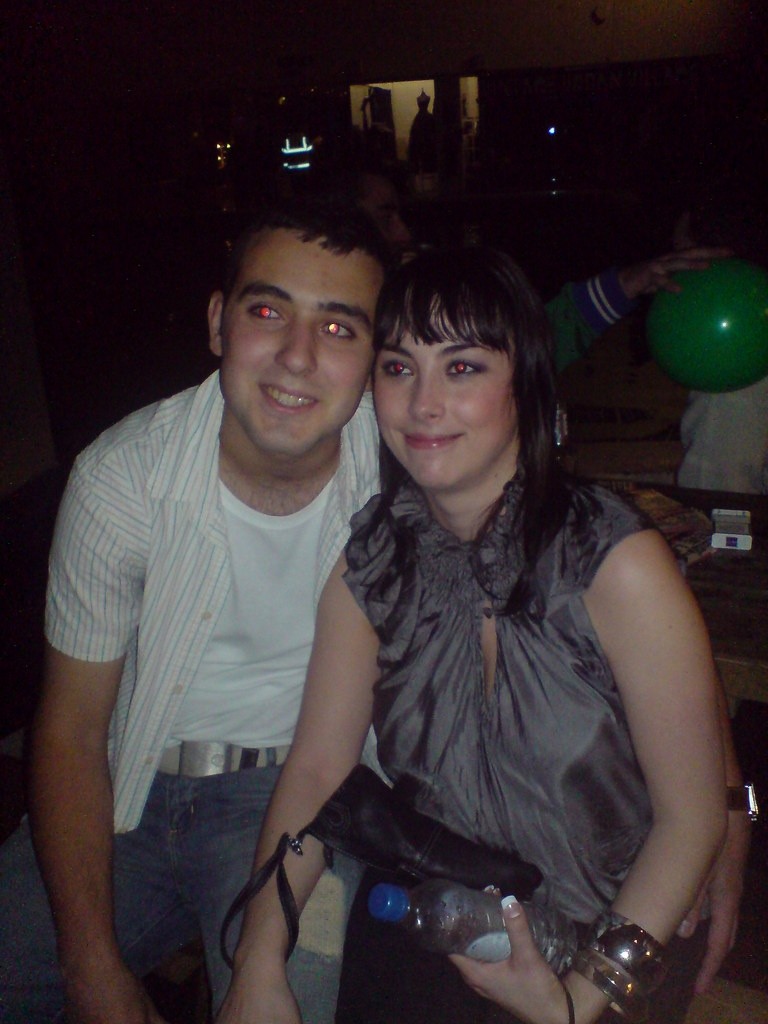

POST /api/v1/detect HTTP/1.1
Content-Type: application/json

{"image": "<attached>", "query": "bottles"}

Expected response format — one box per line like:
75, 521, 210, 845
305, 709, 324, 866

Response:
370, 880, 590, 984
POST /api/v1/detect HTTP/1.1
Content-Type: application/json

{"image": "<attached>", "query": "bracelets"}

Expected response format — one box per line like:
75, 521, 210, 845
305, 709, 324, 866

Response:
569, 908, 676, 1024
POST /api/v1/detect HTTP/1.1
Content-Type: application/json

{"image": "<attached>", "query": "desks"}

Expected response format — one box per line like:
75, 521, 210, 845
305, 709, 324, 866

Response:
637, 488, 768, 719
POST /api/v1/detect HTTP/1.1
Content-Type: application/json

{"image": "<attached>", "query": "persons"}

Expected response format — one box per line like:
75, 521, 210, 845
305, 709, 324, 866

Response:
213, 234, 730, 1024
0, 197, 401, 1024
543, 246, 736, 372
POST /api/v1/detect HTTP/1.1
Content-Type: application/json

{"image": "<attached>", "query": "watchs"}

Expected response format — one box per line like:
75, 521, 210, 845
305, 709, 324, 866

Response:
726, 779, 760, 822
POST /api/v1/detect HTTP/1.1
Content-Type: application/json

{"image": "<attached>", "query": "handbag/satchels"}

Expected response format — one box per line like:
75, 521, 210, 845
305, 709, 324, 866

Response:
218, 765, 544, 970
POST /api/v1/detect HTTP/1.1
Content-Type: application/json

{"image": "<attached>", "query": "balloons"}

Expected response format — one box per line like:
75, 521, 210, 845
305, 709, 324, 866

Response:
636, 254, 768, 393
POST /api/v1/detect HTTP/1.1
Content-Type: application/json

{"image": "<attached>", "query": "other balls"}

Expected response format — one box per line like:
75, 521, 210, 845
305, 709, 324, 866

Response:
646, 257, 768, 394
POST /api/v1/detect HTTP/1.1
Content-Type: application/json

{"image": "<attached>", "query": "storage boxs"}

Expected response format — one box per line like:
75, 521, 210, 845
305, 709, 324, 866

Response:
712, 508, 752, 552
